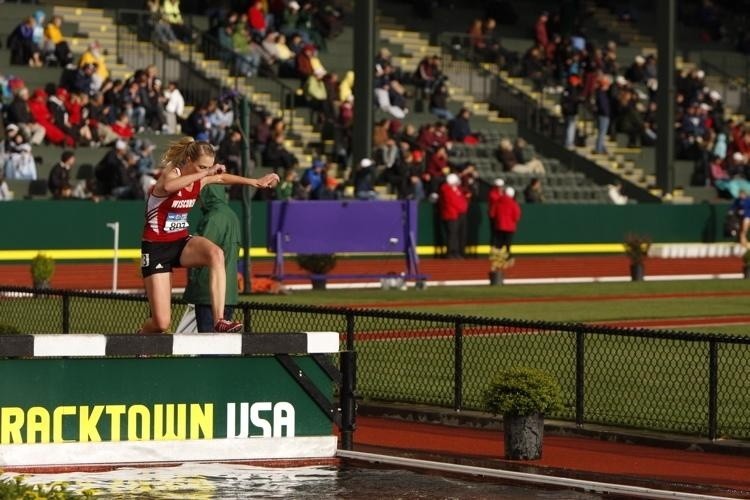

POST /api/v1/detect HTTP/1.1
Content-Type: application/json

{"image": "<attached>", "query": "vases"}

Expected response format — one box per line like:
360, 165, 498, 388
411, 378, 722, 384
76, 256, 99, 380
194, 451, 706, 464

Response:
630, 263, 645, 280
490, 273, 504, 284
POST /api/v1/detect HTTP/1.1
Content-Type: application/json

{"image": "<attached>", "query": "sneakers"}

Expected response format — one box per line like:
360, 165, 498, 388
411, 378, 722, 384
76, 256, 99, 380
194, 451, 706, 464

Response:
213, 318, 243, 333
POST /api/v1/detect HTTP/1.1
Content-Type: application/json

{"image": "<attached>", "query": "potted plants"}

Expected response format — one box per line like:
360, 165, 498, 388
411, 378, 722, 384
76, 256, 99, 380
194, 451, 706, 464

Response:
30, 255, 55, 297
481, 369, 561, 461
298, 251, 333, 290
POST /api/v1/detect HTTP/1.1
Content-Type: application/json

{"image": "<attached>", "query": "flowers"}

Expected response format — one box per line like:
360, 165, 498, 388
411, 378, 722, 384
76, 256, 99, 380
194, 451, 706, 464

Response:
625, 231, 651, 265
490, 246, 515, 272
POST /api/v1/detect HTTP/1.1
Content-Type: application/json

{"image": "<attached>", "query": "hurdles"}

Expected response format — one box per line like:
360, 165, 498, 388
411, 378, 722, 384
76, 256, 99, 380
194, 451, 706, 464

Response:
0, 331, 365, 466
